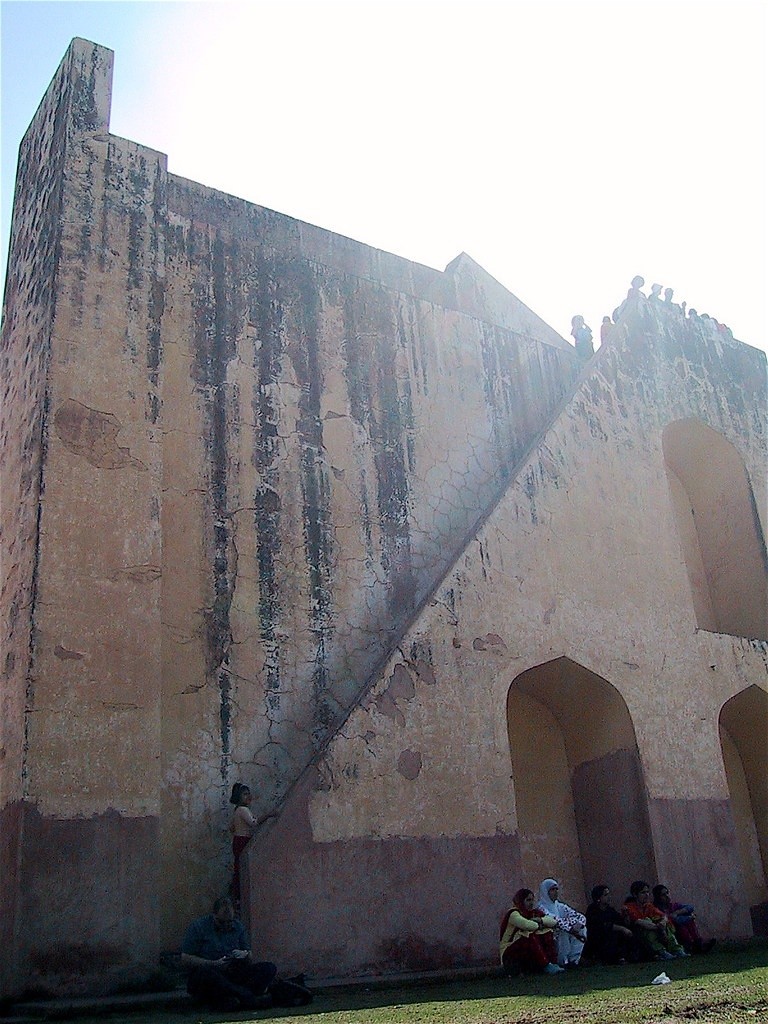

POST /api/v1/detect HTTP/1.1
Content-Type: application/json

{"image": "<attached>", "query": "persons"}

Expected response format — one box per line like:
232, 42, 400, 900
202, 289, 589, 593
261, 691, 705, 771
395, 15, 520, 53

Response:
178, 897, 276, 1012
229, 783, 280, 906
499, 878, 703, 975
570, 276, 697, 361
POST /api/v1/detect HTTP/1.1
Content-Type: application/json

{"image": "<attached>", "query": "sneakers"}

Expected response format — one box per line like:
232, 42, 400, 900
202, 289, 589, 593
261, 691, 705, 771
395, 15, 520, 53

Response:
543, 964, 564, 974
675, 951, 691, 959
655, 952, 677, 959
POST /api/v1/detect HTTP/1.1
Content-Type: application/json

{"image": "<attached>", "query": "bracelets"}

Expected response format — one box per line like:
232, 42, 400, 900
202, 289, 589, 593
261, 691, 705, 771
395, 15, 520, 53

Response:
244, 950, 251, 958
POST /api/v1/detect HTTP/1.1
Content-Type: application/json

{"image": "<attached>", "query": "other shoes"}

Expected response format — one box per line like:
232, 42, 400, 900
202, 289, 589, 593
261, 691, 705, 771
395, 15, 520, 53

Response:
220, 997, 240, 1010
255, 993, 272, 1009
700, 939, 716, 953
